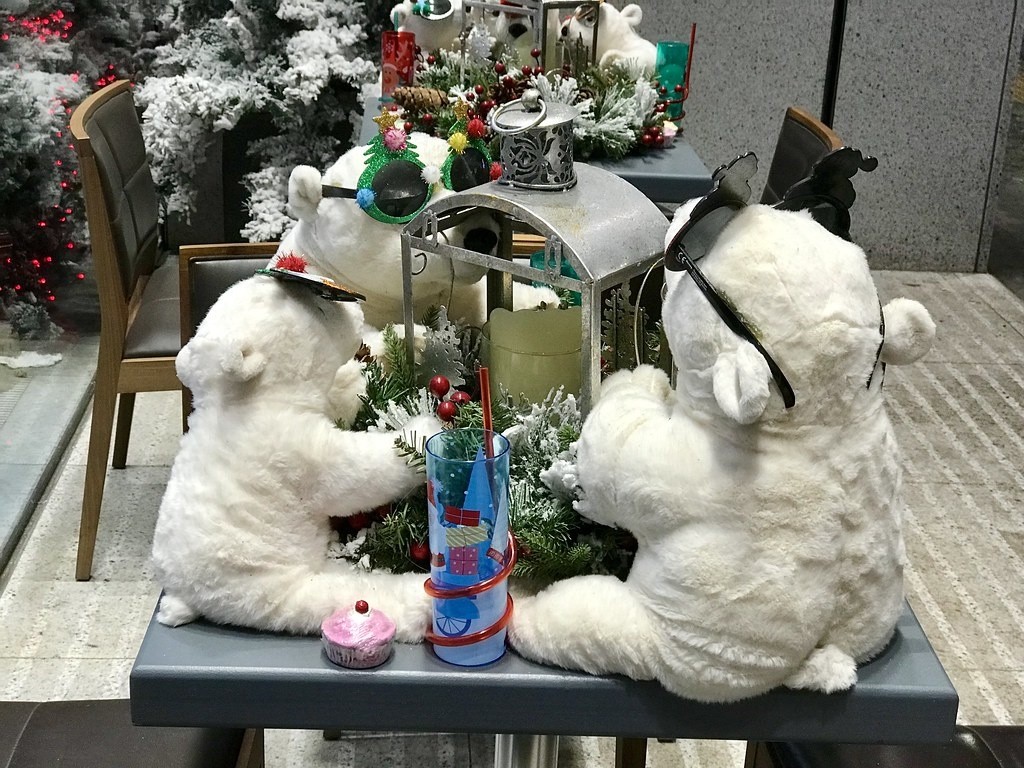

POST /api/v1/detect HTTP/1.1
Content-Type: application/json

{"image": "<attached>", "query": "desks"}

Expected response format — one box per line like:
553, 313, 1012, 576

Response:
359, 98, 713, 331
130, 588, 959, 768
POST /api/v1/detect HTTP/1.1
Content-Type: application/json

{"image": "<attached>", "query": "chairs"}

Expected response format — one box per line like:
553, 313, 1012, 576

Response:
603, 106, 845, 336
70, 79, 179, 581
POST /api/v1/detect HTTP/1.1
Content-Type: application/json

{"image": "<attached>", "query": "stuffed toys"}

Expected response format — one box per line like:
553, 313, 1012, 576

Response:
503, 187, 932, 705
262, 122, 562, 358
150, 263, 444, 638
382, 0, 660, 88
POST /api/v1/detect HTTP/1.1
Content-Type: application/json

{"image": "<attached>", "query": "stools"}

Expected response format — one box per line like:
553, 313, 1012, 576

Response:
0, 700, 264, 768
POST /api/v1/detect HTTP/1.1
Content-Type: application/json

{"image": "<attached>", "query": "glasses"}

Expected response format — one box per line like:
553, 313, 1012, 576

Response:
665, 145, 886, 410
322, 106, 502, 224
259, 253, 367, 303
575, 0, 605, 19
410, 0, 454, 21
